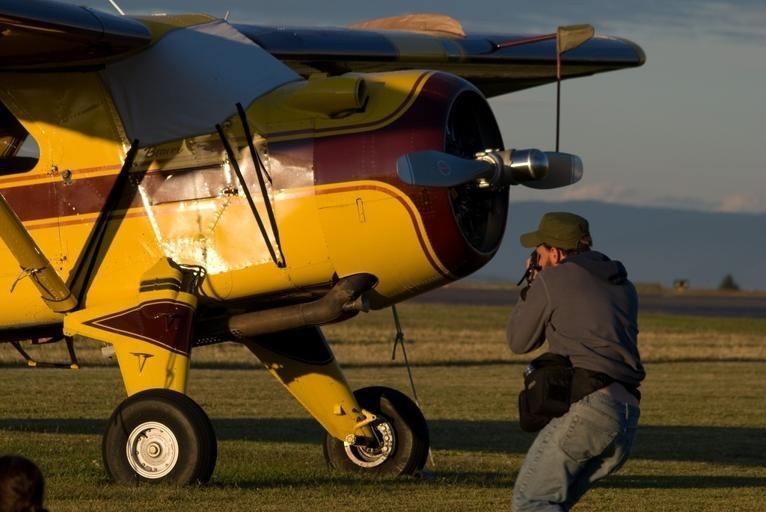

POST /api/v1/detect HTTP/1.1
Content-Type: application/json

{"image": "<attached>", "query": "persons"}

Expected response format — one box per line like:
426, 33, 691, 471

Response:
1, 454, 49, 511
505, 211, 646, 512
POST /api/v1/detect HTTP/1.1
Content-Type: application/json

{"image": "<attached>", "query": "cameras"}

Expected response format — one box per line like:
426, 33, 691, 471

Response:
529, 249, 541, 272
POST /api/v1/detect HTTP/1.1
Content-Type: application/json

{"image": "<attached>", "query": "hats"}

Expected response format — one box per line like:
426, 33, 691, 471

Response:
518, 210, 592, 252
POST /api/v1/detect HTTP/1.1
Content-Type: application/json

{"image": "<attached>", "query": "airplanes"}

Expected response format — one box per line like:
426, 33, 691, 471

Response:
0, 0, 643, 492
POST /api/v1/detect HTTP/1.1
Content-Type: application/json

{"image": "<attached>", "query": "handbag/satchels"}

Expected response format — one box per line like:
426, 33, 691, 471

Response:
509, 373, 574, 428
